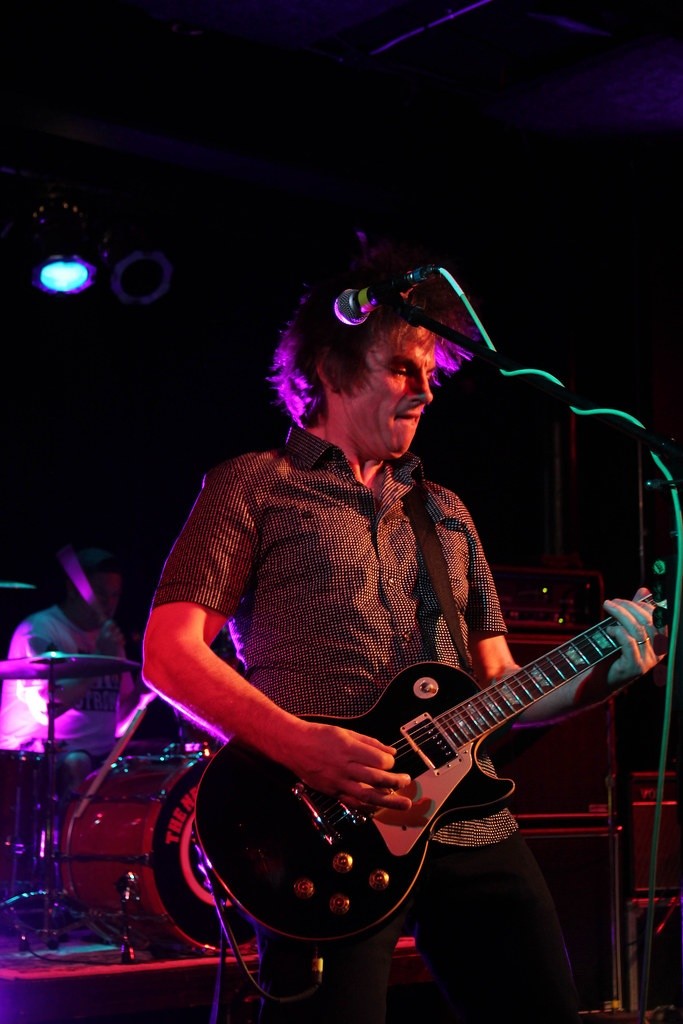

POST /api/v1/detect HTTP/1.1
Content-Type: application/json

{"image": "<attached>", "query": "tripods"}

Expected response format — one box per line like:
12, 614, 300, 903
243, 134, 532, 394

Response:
0, 662, 129, 947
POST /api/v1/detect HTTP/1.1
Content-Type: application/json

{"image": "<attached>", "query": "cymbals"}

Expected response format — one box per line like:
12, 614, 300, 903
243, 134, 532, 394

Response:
0, 650, 141, 680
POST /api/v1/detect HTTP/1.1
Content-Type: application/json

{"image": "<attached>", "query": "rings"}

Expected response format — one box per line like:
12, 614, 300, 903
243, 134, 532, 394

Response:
637, 637, 651, 646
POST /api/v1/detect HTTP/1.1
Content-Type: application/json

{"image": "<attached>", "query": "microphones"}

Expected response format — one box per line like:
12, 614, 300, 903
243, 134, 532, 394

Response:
334, 265, 438, 324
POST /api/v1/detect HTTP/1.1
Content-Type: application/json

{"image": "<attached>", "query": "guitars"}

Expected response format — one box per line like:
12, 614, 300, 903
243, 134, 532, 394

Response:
191, 592, 675, 941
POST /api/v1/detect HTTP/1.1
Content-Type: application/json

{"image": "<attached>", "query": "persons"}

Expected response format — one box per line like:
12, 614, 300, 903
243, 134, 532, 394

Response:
0, 533, 138, 788
140, 245, 671, 1023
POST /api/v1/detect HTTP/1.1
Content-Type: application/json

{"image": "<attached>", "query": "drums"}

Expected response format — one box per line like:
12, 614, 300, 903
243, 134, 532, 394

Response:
58, 751, 259, 956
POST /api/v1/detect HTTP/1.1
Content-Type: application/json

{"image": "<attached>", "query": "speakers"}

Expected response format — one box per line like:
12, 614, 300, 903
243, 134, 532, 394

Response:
491, 630, 680, 1019
